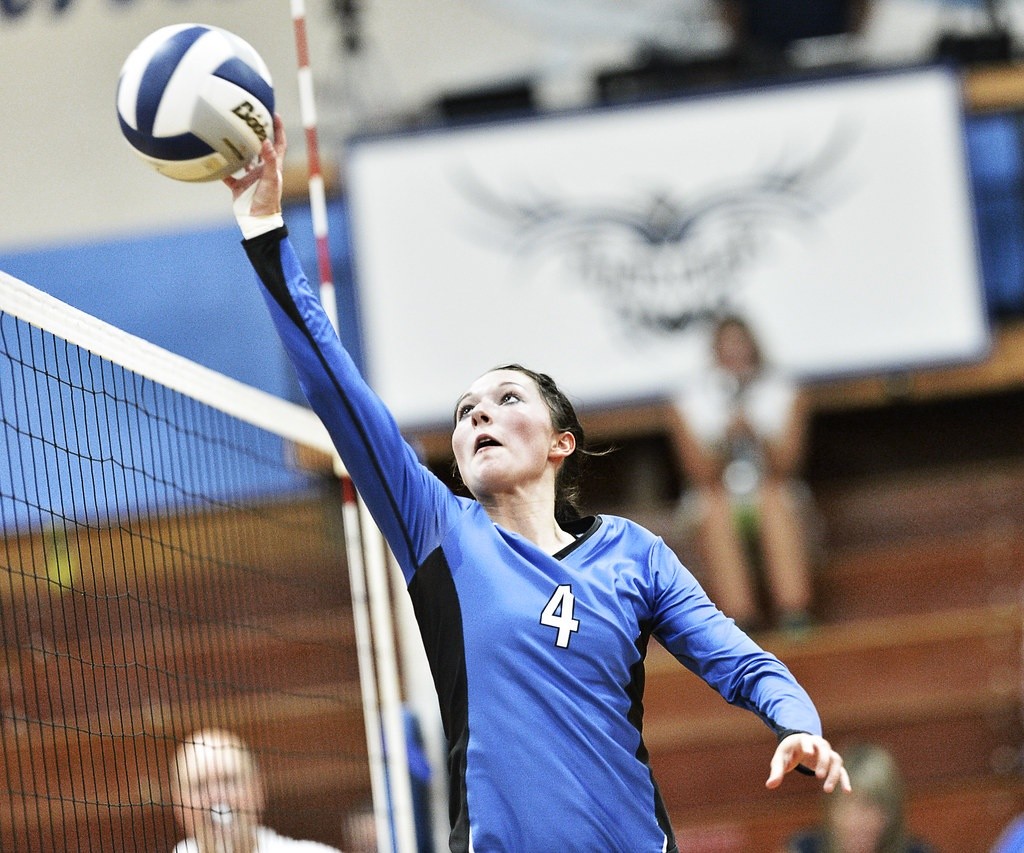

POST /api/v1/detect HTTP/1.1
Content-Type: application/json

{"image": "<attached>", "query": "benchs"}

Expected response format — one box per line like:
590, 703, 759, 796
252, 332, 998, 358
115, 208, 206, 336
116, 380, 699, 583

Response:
0, 465, 1024, 853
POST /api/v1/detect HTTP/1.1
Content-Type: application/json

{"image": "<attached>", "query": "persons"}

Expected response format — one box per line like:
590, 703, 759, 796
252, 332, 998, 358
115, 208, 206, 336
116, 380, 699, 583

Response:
210, 117, 852, 853
664, 315, 827, 629
784, 735, 932, 853
167, 727, 344, 853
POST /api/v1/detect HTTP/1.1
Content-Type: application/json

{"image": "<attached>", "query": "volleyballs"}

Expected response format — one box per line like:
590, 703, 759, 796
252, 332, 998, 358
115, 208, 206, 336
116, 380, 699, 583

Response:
112, 21, 277, 186
